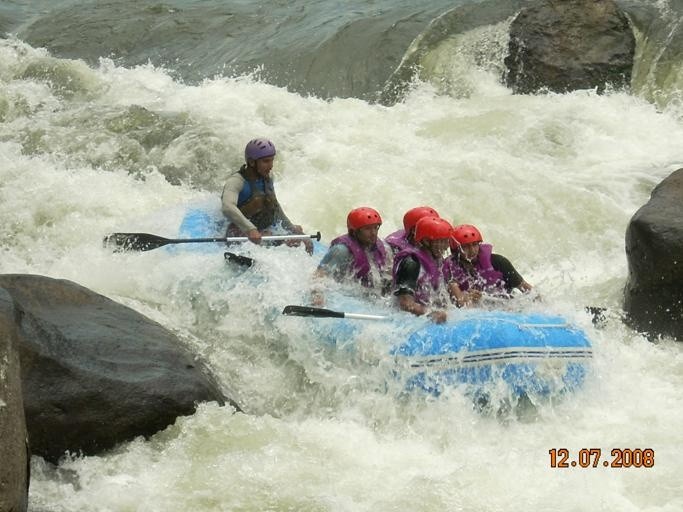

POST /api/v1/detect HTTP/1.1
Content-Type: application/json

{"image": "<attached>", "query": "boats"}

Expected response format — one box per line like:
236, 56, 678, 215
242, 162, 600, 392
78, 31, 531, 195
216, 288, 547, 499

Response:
168, 201, 604, 425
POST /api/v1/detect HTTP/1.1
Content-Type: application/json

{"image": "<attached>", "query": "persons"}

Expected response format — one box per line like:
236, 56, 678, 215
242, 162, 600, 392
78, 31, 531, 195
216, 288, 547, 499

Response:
217, 136, 315, 257
382, 201, 442, 257
440, 224, 542, 313
388, 216, 454, 325
306, 204, 396, 308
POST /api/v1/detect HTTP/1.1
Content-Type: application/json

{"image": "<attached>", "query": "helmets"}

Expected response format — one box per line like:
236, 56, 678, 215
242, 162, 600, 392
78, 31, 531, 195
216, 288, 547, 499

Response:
346, 208, 381, 232
245, 138, 275, 162
403, 207, 453, 246
448, 225, 482, 251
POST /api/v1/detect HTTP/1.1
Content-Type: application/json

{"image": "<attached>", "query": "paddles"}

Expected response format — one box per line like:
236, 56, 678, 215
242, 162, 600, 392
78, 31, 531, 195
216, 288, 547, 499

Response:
104, 233, 321, 253
281, 305, 427, 321
